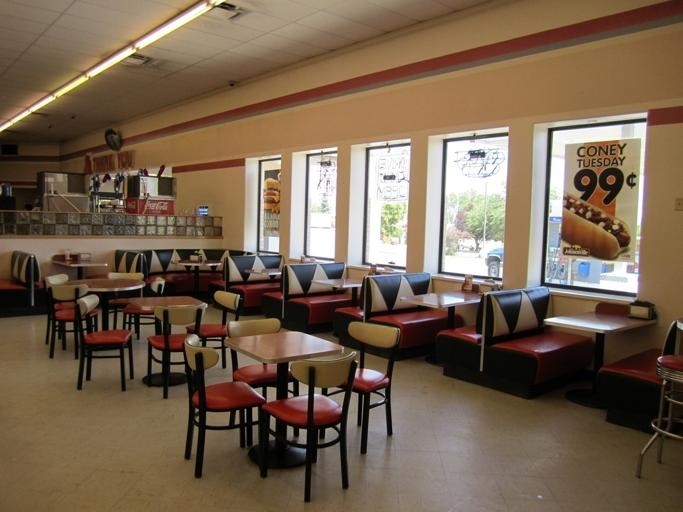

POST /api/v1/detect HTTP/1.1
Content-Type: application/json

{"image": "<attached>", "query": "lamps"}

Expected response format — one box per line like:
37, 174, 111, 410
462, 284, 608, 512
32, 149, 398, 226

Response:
0, 0, 214, 133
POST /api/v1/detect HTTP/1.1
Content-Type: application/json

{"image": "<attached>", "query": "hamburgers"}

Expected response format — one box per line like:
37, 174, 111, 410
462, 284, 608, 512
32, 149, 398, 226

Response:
264, 178, 280, 214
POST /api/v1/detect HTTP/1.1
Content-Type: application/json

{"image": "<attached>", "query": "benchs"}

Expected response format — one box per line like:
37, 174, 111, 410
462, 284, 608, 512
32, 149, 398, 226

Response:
598, 318, 682, 428
227, 257, 359, 330
335, 271, 592, 398
0, 250, 269, 317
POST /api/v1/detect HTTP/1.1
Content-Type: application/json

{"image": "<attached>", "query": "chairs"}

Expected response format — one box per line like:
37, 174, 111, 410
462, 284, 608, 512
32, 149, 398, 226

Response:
46, 273, 243, 400
259, 350, 358, 502
227, 318, 299, 445
318, 321, 400, 455
182, 334, 268, 478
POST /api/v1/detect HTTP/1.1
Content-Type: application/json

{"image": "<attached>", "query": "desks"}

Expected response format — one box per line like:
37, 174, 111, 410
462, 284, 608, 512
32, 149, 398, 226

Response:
225, 332, 344, 470
62, 278, 145, 331
401, 290, 483, 368
124, 297, 200, 388
542, 311, 656, 410
310, 278, 361, 336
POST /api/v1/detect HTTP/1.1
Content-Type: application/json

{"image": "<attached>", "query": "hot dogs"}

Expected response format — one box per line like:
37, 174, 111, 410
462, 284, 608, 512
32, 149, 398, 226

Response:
562, 192, 630, 259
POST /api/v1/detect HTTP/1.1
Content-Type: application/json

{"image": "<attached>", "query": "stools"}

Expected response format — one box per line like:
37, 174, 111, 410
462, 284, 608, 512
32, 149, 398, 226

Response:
636, 355, 683, 478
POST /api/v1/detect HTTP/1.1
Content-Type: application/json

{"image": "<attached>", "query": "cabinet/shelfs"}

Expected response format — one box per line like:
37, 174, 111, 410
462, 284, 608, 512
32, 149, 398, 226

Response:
37, 170, 90, 212
